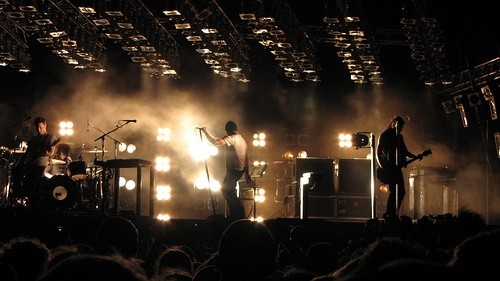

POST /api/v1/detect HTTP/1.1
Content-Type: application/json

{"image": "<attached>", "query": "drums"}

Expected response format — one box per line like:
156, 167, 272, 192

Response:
69, 161, 87, 181
43, 175, 80, 211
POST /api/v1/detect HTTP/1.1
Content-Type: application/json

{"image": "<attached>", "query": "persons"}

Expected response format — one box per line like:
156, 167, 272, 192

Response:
16, 117, 55, 185
51, 144, 72, 174
376, 116, 418, 221
0, 206, 500, 281
202, 121, 255, 220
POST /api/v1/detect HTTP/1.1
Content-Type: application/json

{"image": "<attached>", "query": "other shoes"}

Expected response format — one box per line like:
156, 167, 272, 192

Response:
382, 212, 393, 220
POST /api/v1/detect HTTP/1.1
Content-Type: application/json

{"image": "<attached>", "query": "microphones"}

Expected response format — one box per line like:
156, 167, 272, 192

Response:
123, 120, 137, 122
87, 119, 90, 132
24, 117, 32, 121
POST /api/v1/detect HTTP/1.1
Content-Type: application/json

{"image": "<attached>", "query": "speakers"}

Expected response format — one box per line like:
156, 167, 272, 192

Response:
294, 196, 336, 218
359, 131, 372, 147
292, 158, 335, 195
337, 158, 376, 196
336, 197, 377, 219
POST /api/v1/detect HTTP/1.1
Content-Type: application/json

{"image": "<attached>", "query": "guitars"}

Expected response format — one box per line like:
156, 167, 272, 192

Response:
377, 149, 432, 185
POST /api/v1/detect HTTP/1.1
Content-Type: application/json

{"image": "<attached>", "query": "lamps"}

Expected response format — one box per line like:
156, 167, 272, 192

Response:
398, 2, 461, 86
0, 0, 386, 85
